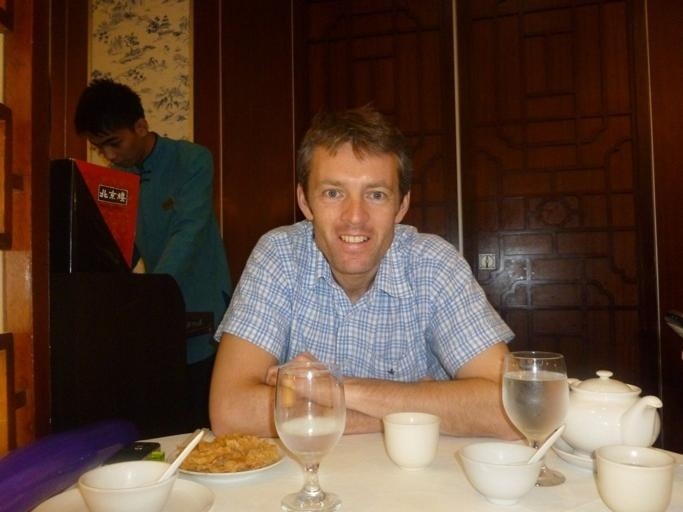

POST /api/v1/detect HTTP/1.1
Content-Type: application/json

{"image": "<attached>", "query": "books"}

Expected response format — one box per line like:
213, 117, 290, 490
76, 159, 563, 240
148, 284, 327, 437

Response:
69, 160, 142, 273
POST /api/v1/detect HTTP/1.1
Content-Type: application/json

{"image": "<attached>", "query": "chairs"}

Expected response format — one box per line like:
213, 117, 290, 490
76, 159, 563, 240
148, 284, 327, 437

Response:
48, 272, 186, 441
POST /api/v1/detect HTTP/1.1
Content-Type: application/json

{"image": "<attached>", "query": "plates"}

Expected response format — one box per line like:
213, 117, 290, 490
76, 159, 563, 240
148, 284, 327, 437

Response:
551, 438, 593, 471
165, 448, 285, 480
31, 478, 216, 512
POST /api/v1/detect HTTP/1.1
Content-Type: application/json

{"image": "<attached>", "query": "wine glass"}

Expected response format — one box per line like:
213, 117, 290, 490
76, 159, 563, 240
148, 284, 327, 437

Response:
270, 360, 345, 511
497, 348, 569, 490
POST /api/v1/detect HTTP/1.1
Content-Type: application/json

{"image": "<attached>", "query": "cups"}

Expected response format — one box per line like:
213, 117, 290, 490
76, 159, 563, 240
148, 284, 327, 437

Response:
379, 410, 441, 471
593, 443, 676, 512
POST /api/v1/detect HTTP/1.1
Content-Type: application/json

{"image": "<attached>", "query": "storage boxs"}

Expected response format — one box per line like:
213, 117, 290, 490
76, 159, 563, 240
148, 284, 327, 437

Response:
50, 157, 140, 275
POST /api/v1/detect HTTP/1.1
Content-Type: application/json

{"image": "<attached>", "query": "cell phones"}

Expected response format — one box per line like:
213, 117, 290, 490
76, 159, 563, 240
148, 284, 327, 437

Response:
102, 442, 160, 464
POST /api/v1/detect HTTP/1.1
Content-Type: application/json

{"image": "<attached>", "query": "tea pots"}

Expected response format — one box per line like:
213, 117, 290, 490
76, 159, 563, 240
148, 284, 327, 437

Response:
566, 367, 666, 456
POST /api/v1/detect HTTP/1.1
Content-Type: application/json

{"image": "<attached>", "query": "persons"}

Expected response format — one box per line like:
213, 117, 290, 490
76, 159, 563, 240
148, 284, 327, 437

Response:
70, 77, 232, 434
204, 103, 531, 442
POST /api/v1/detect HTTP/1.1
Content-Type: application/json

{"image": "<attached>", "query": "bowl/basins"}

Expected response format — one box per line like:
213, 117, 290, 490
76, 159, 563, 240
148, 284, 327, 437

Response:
457, 442, 543, 503
77, 459, 177, 512
